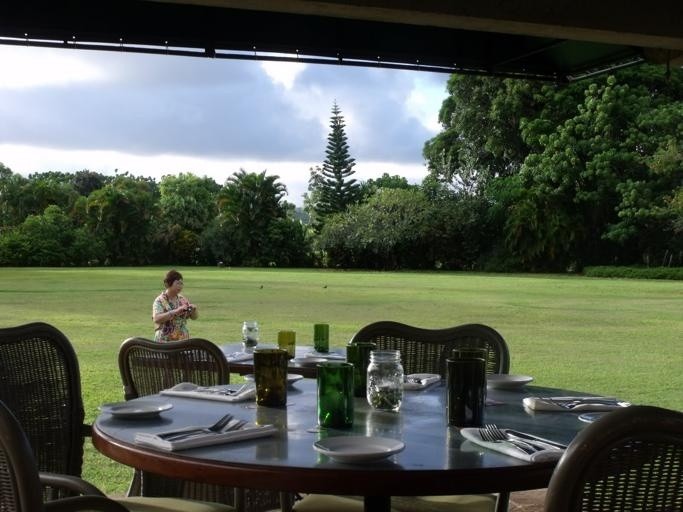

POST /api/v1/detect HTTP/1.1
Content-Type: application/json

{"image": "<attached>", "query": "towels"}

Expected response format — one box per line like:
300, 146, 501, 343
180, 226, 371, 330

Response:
160, 382, 257, 403
402, 373, 441, 390
223, 352, 255, 363
461, 428, 567, 464
135, 419, 275, 453
524, 396, 631, 411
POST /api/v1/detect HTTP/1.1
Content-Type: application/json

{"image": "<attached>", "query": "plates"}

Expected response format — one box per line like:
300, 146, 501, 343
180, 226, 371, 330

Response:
577, 411, 613, 423
287, 374, 303, 383
98, 402, 174, 419
289, 357, 328, 367
486, 375, 534, 389
313, 436, 406, 463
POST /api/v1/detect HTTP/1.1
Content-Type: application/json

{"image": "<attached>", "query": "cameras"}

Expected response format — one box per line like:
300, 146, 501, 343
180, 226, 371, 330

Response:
184, 306, 193, 312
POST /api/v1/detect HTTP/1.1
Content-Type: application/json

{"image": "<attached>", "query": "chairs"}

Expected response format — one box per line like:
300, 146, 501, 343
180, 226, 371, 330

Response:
0, 402, 126, 511
292, 322, 510, 512
0, 322, 234, 512
542, 406, 683, 511
118, 337, 292, 512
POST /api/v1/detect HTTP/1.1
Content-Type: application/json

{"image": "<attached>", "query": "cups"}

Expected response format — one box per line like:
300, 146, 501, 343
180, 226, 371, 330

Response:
242, 322, 258, 348
314, 324, 329, 353
277, 332, 297, 357
451, 347, 488, 410
316, 361, 355, 430
366, 350, 404, 412
346, 342, 377, 399
445, 357, 486, 428
253, 349, 288, 407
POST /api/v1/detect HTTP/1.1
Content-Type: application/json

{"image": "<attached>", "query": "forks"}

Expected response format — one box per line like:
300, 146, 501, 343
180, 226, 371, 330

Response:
406, 377, 432, 384
156, 413, 234, 441
484, 424, 546, 452
479, 428, 532, 455
168, 420, 248, 443
540, 398, 619, 410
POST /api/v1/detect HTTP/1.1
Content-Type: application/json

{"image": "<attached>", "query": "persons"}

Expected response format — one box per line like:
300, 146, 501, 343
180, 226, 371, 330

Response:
152, 271, 198, 344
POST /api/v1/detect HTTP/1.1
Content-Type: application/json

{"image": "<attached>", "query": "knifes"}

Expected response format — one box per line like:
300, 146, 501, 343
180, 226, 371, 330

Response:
505, 429, 568, 450
